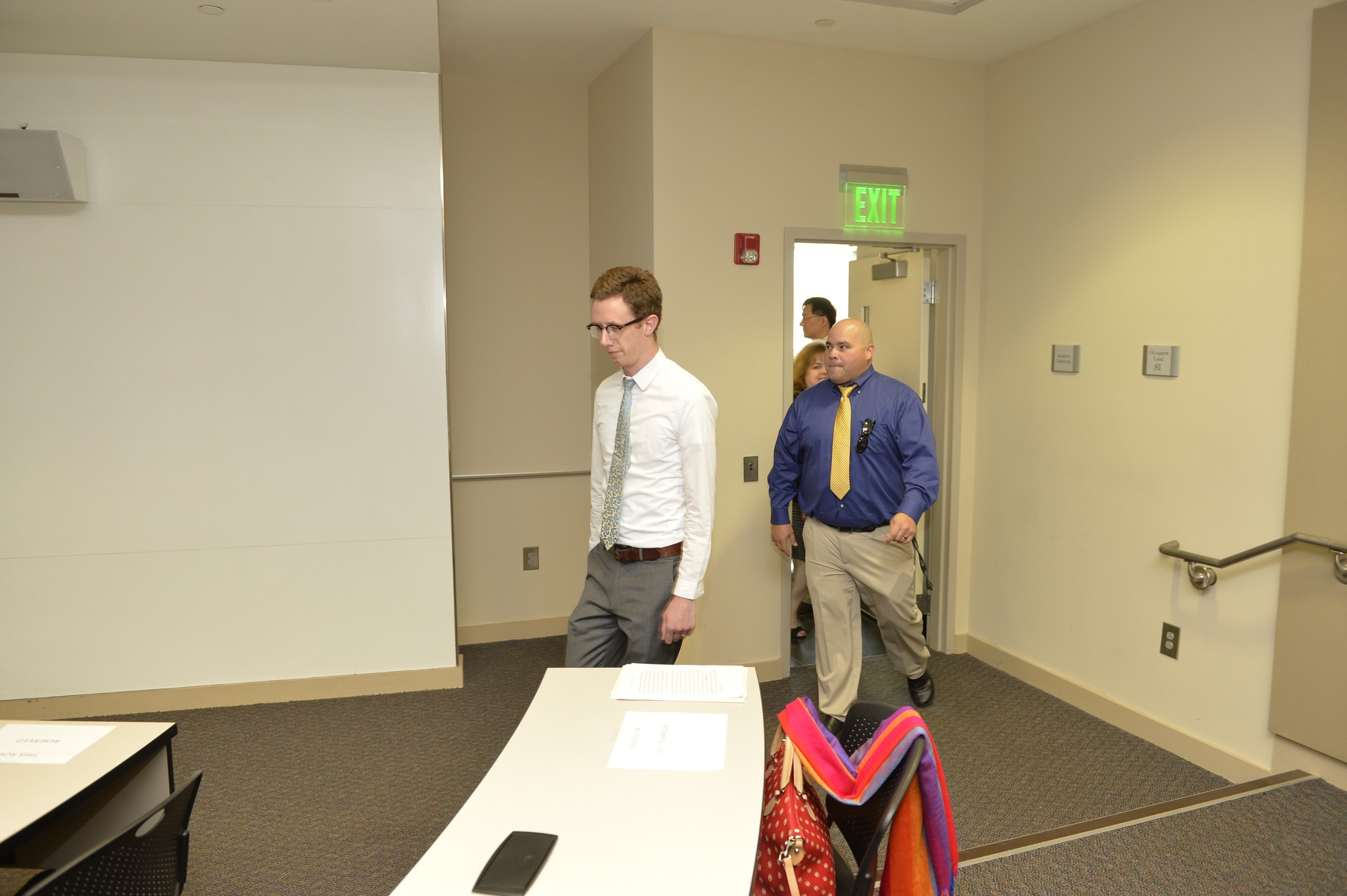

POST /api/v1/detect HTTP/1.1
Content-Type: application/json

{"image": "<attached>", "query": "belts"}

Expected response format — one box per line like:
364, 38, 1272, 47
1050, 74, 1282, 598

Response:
821, 523, 890, 533
604, 542, 683, 562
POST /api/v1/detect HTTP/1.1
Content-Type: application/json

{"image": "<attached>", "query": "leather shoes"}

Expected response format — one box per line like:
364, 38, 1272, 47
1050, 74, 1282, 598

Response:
823, 715, 843, 738
907, 668, 934, 707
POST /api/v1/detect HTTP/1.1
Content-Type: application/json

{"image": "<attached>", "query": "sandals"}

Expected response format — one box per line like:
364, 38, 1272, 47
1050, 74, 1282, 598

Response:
792, 626, 807, 640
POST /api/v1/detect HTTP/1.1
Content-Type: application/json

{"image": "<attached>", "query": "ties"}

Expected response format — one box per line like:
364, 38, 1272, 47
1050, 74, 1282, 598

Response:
601, 377, 635, 550
831, 383, 858, 500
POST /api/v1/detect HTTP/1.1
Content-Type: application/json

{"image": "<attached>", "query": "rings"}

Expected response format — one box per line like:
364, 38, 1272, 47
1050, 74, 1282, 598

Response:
903, 536, 907, 540
681, 634, 688, 637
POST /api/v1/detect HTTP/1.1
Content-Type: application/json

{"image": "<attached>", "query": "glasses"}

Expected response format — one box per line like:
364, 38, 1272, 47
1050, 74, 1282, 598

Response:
857, 418, 876, 453
586, 317, 645, 340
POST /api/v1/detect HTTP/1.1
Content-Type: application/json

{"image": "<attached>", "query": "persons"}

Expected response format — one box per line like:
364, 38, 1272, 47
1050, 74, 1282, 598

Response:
564, 266, 720, 669
791, 338, 828, 640
768, 318, 937, 739
791, 297, 837, 613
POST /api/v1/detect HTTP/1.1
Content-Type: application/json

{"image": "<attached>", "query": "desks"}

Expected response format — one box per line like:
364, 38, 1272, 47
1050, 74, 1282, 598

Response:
0, 714, 179, 872
383, 665, 766, 896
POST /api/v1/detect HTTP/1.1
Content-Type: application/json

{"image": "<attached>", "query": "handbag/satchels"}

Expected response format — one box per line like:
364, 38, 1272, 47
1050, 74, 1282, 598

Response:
751, 723, 837, 896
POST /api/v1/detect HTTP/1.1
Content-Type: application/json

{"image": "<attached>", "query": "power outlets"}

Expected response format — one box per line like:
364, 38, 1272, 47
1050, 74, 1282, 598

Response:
523, 546, 539, 571
1159, 621, 1181, 660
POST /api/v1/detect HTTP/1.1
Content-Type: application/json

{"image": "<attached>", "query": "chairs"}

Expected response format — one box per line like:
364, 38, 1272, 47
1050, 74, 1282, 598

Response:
825, 701, 924, 896
20, 770, 203, 895
